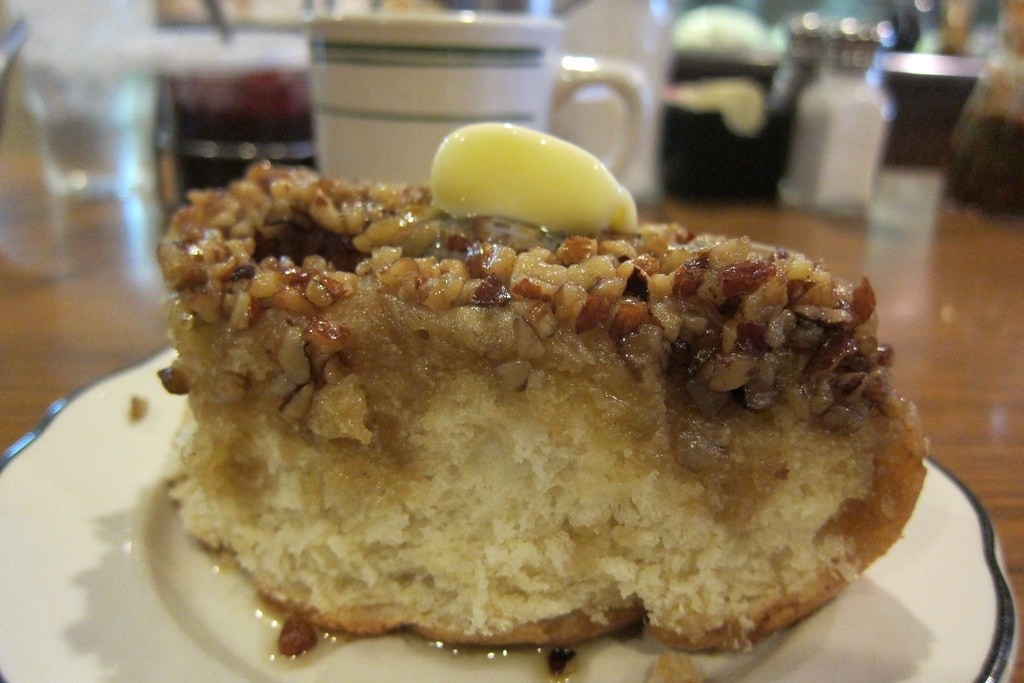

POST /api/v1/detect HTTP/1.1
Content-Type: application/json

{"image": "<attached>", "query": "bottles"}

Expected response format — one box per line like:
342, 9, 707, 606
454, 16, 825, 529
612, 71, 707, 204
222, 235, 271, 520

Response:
769, 19, 840, 187
776, 18, 896, 218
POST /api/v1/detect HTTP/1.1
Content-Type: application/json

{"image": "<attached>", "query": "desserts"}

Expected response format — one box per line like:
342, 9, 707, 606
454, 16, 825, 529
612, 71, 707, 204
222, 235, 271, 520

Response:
157, 113, 927, 649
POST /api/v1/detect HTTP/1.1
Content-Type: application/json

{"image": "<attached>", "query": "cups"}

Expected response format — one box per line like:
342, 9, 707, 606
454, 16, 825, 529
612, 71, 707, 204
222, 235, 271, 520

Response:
658, 44, 783, 195
301, 11, 655, 190
27, 37, 160, 199
153, 34, 313, 210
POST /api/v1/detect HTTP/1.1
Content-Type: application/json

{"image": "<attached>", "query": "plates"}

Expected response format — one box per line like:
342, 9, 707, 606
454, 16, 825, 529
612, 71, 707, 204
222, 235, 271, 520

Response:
1, 335, 1019, 682
